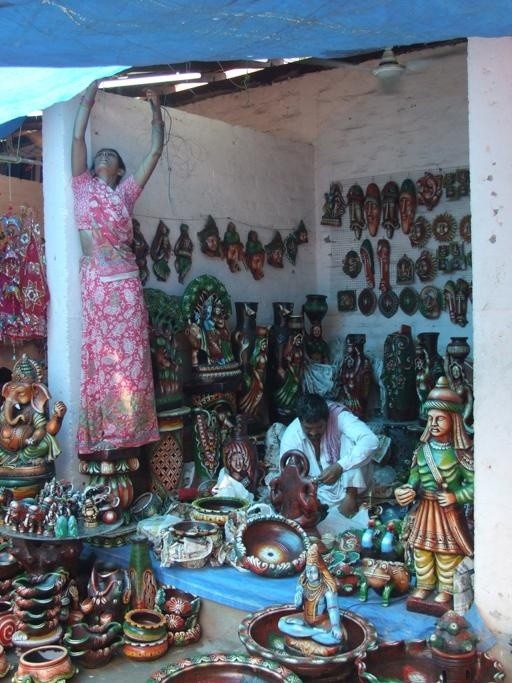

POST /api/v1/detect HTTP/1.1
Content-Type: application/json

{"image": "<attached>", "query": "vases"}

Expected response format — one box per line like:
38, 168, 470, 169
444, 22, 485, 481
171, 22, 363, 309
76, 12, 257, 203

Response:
413, 332, 444, 427
232, 301, 309, 385
304, 294, 328, 322
340, 332, 372, 421
446, 337, 471, 364
161, 495, 312, 578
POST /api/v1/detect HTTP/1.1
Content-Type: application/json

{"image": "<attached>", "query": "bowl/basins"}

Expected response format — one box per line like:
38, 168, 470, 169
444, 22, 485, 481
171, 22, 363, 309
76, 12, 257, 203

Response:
122, 607, 167, 642
234, 513, 310, 575
121, 633, 169, 662
169, 520, 222, 547
146, 650, 303, 683
354, 639, 506, 681
238, 603, 378, 679
191, 496, 251, 524
12, 645, 75, 682
129, 491, 163, 521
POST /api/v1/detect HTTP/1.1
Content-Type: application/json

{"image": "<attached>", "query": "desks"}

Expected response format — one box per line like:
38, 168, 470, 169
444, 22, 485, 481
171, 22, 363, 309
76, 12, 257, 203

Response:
0, 510, 125, 574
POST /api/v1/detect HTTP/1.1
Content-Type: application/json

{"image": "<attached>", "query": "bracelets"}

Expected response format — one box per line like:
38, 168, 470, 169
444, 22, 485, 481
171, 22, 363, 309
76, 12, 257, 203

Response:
151, 118, 165, 127
79, 96, 95, 110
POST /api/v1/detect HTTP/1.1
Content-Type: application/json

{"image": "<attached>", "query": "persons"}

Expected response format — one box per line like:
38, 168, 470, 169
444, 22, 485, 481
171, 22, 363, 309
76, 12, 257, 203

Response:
153, 290, 474, 482
395, 375, 474, 606
278, 544, 343, 645
227, 442, 253, 481
131, 217, 194, 286
279, 393, 379, 516
71, 73, 165, 456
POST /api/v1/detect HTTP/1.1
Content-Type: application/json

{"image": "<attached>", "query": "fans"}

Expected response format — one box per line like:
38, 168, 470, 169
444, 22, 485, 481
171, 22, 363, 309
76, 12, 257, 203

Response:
303, 46, 467, 98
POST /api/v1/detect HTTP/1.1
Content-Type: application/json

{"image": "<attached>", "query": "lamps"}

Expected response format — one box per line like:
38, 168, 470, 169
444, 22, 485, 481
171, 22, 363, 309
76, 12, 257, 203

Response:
97, 63, 202, 90
